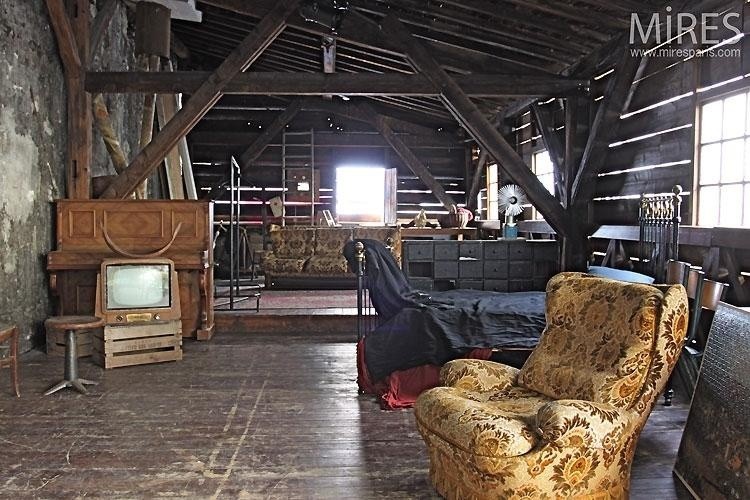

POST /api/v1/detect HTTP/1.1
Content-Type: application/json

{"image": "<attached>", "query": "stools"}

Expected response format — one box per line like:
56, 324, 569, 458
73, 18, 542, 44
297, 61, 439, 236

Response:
41, 315, 104, 400
0, 323, 22, 399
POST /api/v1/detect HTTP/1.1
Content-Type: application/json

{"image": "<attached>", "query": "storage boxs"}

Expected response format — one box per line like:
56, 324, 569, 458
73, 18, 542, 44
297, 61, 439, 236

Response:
45, 325, 94, 361
89, 318, 183, 370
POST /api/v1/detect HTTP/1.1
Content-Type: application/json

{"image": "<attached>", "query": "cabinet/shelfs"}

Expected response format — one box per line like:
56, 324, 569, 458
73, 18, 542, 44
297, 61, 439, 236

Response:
46, 198, 215, 342
470, 143, 487, 220
402, 239, 560, 293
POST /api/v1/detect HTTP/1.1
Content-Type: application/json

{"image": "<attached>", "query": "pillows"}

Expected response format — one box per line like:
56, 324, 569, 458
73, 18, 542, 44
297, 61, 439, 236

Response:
517, 273, 664, 412
587, 264, 655, 288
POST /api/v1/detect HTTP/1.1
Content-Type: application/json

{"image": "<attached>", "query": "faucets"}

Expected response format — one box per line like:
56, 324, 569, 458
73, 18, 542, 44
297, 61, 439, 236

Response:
449, 203, 462, 228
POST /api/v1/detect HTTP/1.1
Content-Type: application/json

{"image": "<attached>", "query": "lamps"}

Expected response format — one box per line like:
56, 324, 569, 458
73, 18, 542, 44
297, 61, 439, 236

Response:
297, 0, 350, 74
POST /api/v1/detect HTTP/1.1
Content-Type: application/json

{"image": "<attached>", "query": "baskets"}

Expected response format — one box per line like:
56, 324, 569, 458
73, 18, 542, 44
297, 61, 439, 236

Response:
437, 204, 468, 228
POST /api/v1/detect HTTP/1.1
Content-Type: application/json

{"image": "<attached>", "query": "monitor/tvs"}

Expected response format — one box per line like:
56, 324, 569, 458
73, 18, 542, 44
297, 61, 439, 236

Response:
95, 257, 182, 324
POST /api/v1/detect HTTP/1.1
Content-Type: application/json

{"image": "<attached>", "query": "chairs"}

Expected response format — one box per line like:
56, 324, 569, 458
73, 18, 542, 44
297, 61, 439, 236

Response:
414, 270, 689, 500
653, 258, 730, 405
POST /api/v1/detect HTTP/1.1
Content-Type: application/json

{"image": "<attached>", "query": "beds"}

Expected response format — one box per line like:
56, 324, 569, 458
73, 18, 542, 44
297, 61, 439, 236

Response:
353, 224, 713, 411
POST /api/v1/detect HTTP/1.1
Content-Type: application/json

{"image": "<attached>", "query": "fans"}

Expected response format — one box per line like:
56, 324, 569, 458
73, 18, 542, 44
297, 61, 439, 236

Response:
494, 183, 527, 239
